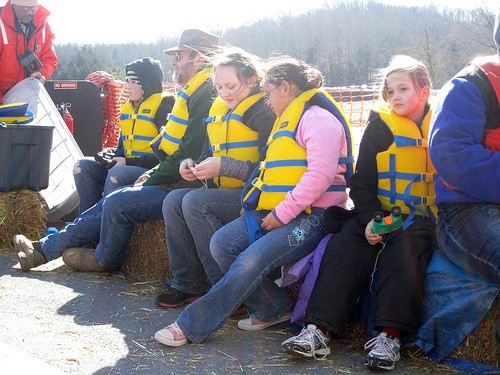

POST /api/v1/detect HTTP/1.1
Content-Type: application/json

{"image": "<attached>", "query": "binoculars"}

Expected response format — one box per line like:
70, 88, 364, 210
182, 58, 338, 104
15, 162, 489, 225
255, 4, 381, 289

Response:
17, 50, 43, 73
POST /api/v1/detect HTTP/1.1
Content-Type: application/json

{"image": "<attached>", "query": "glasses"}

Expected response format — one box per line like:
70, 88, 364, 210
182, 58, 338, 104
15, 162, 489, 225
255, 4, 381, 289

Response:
21, 7, 37, 12
175, 52, 184, 63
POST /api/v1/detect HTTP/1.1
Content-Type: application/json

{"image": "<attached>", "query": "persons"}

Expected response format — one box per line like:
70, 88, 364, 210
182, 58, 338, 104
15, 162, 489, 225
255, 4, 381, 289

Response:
154, 56, 347, 346
282, 54, 438, 370
428, 9, 500, 282
73, 56, 176, 215
0, 0, 59, 106
156, 45, 277, 315
13, 34, 218, 272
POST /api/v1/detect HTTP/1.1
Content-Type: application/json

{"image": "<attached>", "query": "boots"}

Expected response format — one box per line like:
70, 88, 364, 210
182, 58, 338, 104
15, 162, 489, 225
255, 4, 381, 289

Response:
63, 248, 108, 271
13, 234, 45, 272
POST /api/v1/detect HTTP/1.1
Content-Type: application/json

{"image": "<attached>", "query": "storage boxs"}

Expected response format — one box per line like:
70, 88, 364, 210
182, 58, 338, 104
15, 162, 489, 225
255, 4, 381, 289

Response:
0, 125, 55, 192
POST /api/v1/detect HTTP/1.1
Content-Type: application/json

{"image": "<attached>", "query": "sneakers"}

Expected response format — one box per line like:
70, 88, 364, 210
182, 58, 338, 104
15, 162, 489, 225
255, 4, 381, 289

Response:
237, 314, 292, 331
232, 303, 249, 317
280, 325, 331, 360
155, 322, 188, 347
157, 287, 205, 308
364, 334, 400, 370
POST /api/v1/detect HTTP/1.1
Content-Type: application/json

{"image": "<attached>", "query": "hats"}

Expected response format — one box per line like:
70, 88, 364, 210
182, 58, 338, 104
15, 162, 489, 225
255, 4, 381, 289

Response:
493, 8, 500, 52
10, 0, 41, 6
163, 29, 221, 55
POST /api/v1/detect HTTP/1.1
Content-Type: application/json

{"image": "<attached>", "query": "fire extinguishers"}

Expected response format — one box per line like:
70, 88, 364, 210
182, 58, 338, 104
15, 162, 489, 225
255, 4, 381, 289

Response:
57, 102, 74, 135
